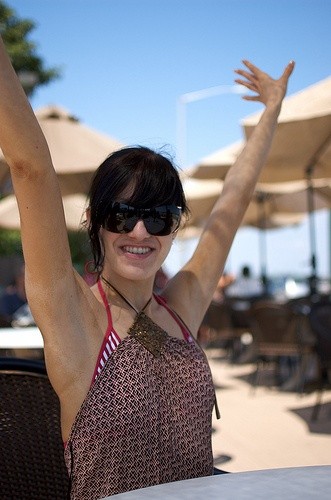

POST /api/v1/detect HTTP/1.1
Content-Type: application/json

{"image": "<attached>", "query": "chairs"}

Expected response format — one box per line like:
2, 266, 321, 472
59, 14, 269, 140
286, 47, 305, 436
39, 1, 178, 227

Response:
0, 367, 76, 500
198, 292, 315, 391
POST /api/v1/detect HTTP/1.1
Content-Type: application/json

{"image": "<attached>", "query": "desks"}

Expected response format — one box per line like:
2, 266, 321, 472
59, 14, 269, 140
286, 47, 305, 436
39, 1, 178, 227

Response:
89, 465, 331, 500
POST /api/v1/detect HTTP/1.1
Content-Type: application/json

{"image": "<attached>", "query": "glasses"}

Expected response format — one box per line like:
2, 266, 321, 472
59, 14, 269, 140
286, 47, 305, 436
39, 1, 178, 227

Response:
94, 201, 182, 235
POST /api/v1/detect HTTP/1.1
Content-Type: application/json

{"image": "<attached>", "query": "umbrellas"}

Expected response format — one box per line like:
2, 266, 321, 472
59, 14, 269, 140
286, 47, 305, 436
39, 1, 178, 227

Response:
177, 137, 331, 182
0, 103, 124, 198
177, 180, 304, 297
1, 193, 92, 231
241, 75, 331, 154
251, 177, 331, 276
171, 225, 203, 241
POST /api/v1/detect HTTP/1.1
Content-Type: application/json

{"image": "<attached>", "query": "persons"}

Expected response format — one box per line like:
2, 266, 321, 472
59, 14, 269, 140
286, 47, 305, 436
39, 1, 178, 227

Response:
225, 266, 266, 348
0, 35, 296, 500
